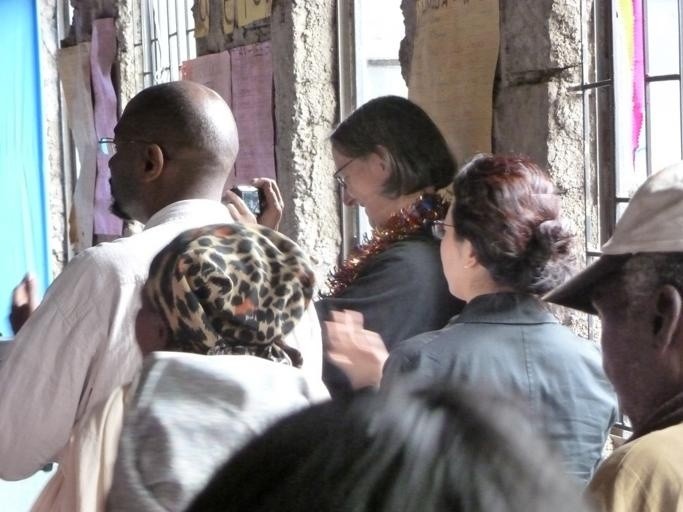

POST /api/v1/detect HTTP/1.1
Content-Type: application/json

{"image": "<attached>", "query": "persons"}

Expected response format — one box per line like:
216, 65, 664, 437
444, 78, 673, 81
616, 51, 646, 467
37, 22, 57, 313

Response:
39, 221, 333, 512
182, 378, 594, 512
0, 78, 324, 483
324, 153, 622, 482
222, 93, 468, 398
541, 159, 683, 512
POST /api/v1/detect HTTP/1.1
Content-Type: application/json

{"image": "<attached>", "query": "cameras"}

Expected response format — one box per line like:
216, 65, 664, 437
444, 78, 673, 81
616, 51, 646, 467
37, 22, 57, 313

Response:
231, 184, 261, 219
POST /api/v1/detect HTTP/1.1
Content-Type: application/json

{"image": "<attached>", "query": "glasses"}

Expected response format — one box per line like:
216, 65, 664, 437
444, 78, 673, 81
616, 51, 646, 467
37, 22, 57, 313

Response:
98, 137, 147, 156
333, 154, 359, 188
430, 220, 456, 240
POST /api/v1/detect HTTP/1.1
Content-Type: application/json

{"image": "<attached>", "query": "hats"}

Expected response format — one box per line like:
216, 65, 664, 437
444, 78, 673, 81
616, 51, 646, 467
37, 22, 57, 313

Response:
540, 160, 683, 317
